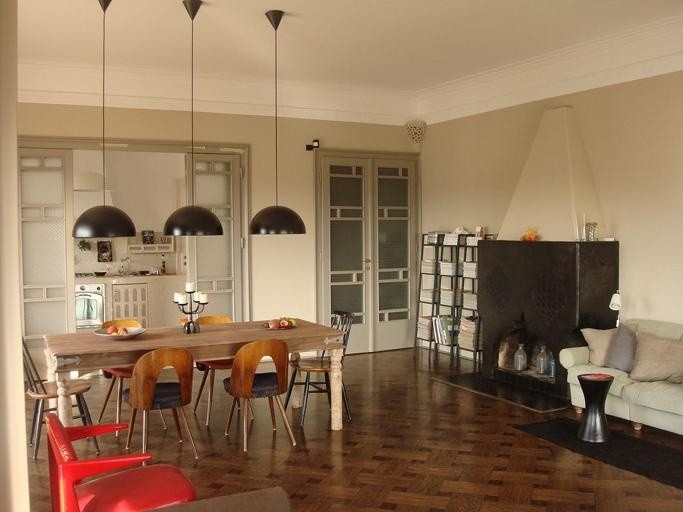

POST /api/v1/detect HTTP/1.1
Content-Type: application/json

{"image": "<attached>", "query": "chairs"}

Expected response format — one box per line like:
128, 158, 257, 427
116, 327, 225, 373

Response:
282, 307, 356, 433
96, 318, 170, 441
192, 313, 256, 432
116, 341, 201, 470
21, 336, 100, 459
222, 338, 302, 454
44, 402, 196, 512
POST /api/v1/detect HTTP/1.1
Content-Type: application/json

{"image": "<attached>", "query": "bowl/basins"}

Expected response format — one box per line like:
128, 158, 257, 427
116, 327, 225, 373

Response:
93, 271, 107, 277
138, 270, 150, 276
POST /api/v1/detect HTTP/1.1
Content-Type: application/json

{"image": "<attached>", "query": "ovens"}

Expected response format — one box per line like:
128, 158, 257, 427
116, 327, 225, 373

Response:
72, 283, 108, 328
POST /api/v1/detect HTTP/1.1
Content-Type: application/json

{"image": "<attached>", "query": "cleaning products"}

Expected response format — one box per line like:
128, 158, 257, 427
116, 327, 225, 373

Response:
536, 346, 548, 375
548, 351, 556, 377
513, 343, 528, 370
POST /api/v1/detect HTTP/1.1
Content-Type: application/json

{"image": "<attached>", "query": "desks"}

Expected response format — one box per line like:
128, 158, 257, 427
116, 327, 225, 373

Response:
572, 371, 618, 446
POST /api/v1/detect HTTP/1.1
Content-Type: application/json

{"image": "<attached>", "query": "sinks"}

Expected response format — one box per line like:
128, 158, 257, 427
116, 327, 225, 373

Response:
94, 270, 140, 278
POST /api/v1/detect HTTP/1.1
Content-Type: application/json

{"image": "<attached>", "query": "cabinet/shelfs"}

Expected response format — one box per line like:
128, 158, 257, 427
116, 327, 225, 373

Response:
108, 272, 183, 326
411, 232, 486, 374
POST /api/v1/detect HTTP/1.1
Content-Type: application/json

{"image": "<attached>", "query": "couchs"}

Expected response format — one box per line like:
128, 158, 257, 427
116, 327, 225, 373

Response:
557, 312, 682, 450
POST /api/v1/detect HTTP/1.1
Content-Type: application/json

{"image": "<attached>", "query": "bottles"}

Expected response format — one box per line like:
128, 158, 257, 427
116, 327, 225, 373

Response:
159, 254, 165, 276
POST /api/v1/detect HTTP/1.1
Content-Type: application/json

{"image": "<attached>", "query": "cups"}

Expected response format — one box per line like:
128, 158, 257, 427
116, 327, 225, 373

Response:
572, 211, 585, 241
585, 221, 598, 242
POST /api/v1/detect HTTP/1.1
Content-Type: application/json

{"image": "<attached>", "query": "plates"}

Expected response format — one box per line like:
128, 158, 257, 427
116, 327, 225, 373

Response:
89, 327, 148, 340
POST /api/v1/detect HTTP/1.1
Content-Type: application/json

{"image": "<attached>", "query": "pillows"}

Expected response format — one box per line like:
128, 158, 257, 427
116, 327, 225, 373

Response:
624, 331, 681, 386
601, 319, 642, 372
577, 324, 636, 370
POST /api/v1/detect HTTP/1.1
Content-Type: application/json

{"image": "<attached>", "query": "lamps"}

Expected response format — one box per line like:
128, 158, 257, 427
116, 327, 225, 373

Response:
304, 137, 320, 151
246, 10, 307, 237
71, 0, 136, 239
163, 0, 225, 238
609, 287, 621, 327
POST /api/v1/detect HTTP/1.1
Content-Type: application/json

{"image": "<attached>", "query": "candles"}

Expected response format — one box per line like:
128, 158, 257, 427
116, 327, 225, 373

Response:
170, 278, 208, 308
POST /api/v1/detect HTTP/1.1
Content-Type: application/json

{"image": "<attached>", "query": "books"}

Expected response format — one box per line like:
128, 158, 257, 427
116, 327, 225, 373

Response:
416, 224, 484, 352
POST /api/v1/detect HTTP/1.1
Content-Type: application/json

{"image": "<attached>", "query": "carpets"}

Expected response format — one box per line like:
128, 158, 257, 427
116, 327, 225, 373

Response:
430, 368, 585, 415
507, 414, 682, 491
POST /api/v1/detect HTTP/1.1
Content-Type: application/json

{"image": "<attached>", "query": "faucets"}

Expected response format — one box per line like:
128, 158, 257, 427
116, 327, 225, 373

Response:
119, 255, 132, 273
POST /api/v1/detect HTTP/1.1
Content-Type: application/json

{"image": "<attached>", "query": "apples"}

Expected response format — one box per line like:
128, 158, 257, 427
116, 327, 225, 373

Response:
269, 319, 280, 328
107, 325, 117, 335
117, 327, 128, 336
